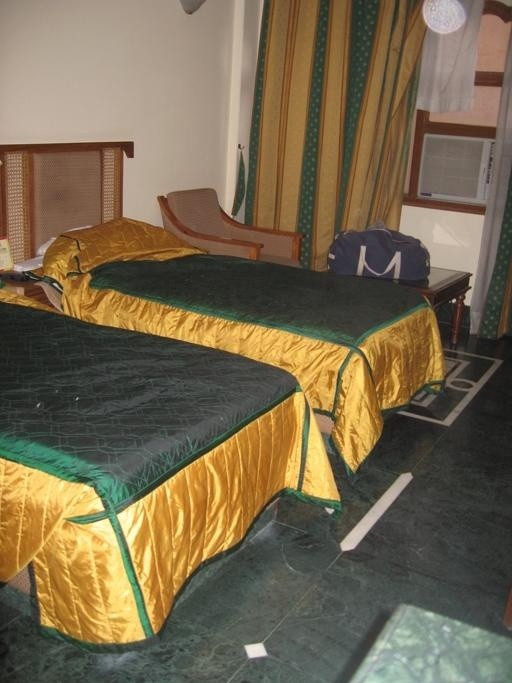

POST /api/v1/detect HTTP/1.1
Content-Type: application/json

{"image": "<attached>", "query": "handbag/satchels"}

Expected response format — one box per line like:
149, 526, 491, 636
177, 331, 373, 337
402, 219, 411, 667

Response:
327, 229, 430, 281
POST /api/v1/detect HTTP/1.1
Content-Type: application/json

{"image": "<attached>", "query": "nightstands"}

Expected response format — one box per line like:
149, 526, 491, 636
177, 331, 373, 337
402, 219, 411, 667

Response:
6, 276, 55, 310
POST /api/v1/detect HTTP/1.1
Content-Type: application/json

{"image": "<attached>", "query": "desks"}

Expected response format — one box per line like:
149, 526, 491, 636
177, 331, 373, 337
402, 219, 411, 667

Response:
347, 601, 512, 682
405, 265, 474, 349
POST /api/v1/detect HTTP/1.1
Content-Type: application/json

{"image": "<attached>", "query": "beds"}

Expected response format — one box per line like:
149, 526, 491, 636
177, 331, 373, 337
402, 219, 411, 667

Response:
1, 298, 336, 655
1, 141, 466, 479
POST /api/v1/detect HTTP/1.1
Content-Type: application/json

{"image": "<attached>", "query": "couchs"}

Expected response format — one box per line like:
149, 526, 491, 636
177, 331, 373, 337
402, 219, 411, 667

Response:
158, 188, 304, 265
